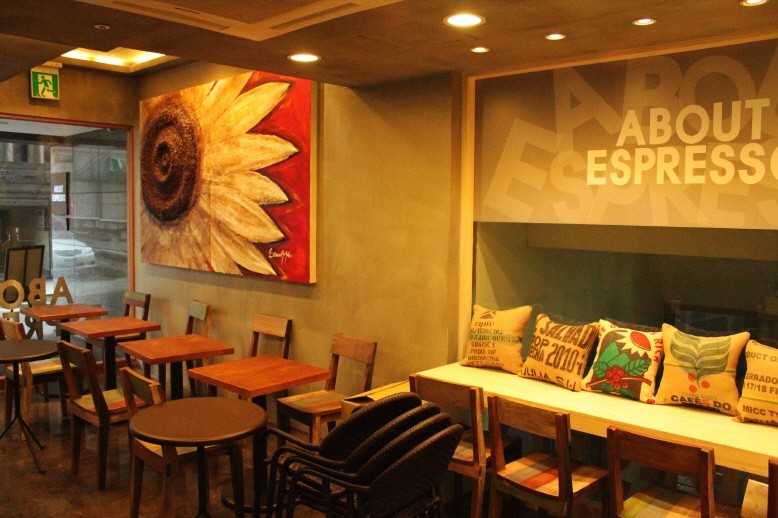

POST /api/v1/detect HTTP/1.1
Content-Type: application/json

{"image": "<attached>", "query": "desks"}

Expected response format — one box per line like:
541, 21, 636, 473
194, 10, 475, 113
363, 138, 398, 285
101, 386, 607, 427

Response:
127, 397, 266, 518
50, 315, 159, 421
0, 335, 67, 474
411, 360, 778, 518
24, 303, 101, 403
183, 350, 336, 510
109, 334, 233, 400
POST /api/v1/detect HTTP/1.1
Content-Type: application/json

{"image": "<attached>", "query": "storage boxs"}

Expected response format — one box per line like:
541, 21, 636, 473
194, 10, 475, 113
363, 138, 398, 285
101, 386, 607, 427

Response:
341, 376, 415, 426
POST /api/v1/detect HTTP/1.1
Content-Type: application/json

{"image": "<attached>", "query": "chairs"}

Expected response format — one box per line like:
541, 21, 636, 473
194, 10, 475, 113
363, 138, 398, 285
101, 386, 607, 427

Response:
0, 291, 778, 518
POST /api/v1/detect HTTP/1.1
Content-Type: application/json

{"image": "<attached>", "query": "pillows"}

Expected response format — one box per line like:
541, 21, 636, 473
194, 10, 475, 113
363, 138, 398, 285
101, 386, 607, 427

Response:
518, 312, 600, 391
733, 338, 778, 425
458, 303, 534, 374
655, 323, 750, 415
579, 319, 669, 405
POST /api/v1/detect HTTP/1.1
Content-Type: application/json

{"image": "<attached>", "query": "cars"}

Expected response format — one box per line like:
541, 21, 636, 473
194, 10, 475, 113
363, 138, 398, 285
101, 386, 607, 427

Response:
42, 213, 98, 281
